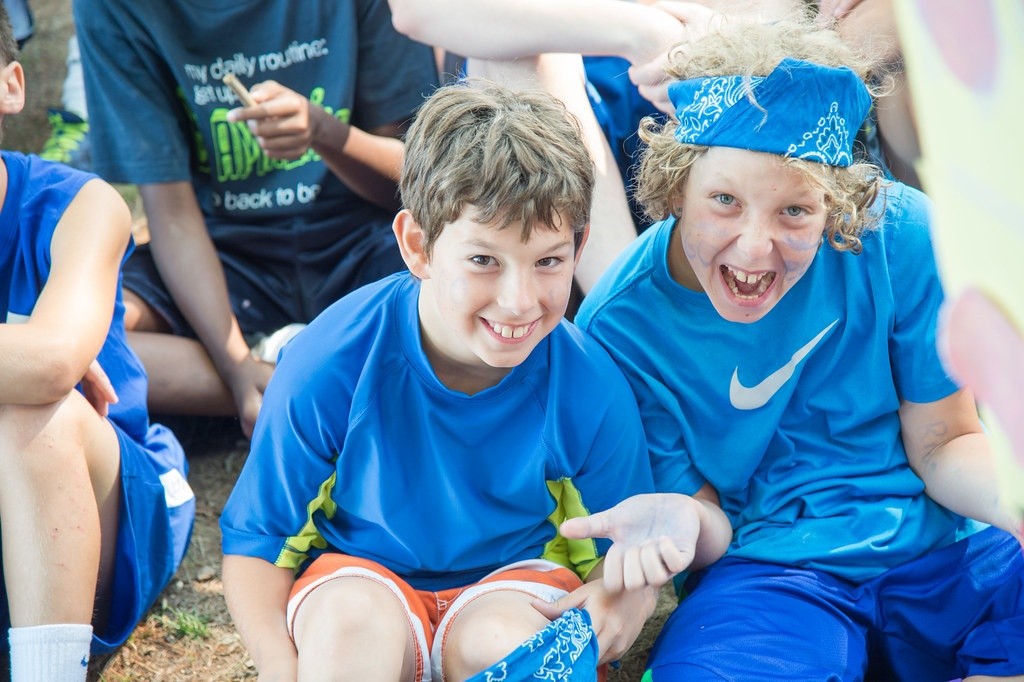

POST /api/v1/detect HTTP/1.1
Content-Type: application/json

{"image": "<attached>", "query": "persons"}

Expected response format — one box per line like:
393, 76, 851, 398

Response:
385, 1, 925, 310
565, 14, 1023, 681
0, 0, 200, 680
54, 0, 442, 454
214, 83, 655, 682
